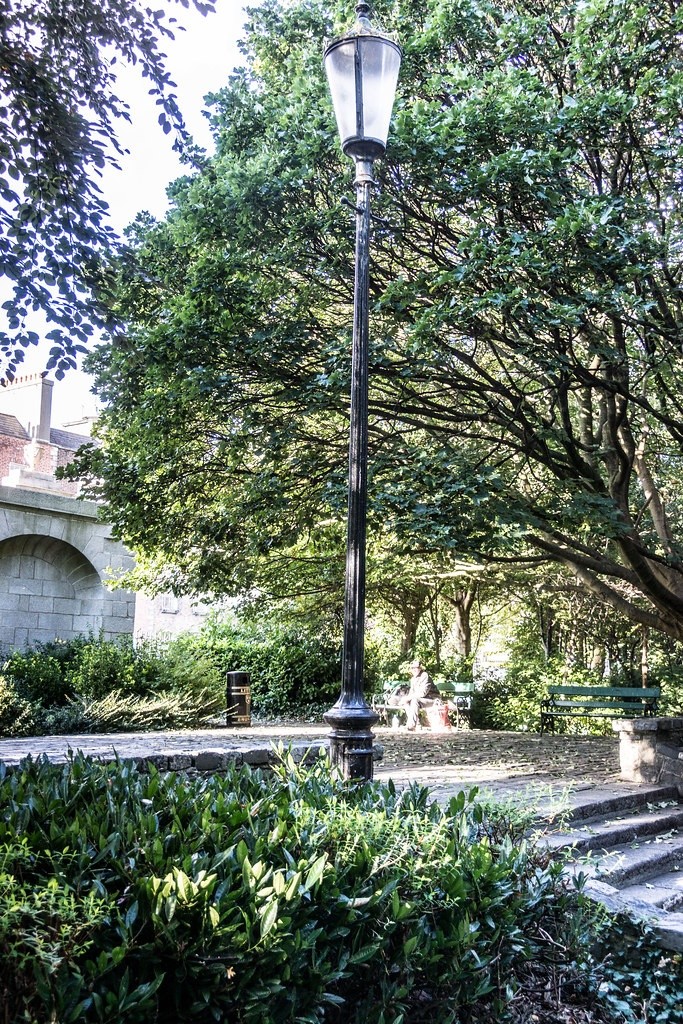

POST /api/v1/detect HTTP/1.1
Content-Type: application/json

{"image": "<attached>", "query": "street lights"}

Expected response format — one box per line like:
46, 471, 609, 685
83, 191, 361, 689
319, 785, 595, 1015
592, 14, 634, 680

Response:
321, 2, 402, 807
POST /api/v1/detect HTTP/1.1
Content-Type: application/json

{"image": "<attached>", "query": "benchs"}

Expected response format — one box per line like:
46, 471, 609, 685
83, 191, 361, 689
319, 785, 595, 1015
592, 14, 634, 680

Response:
539, 685, 661, 737
371, 681, 475, 731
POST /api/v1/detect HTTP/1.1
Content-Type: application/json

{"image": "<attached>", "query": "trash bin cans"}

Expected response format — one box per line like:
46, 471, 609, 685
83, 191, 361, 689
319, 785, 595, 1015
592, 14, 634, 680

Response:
226, 671, 251, 727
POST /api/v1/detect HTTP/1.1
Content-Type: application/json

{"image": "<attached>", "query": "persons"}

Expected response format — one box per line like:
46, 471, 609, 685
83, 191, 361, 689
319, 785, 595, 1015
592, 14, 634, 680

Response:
399, 657, 441, 731
